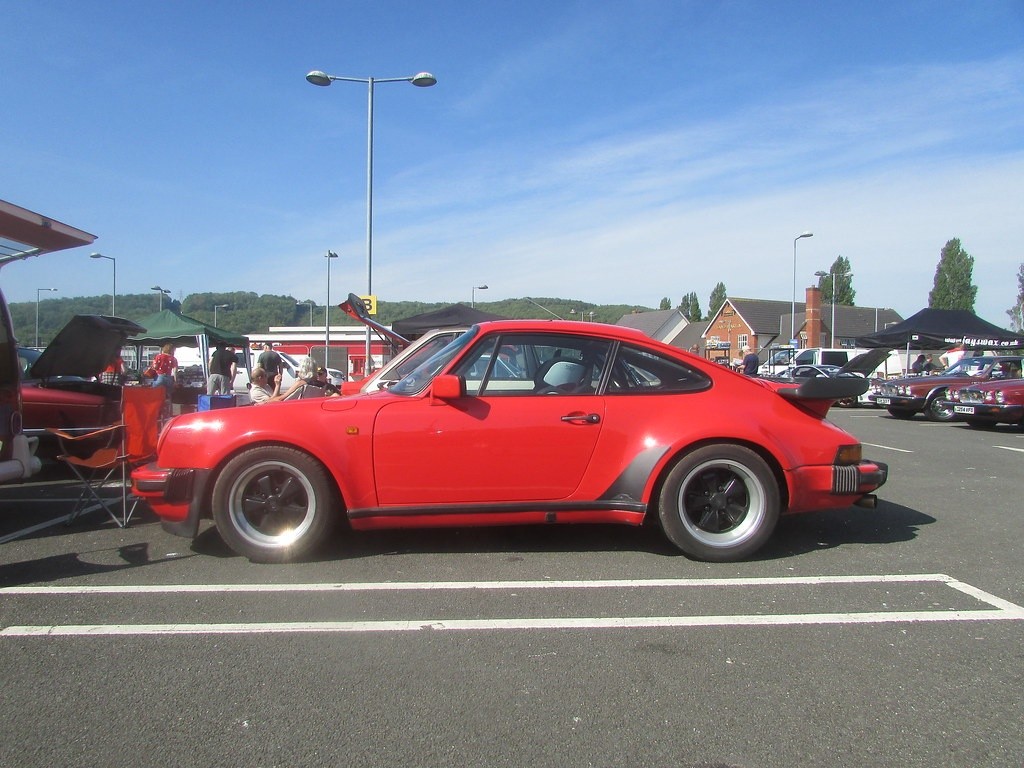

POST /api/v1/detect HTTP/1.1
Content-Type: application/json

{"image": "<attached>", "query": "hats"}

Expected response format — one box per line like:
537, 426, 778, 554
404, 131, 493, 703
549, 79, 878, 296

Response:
317, 367, 327, 373
264, 342, 272, 346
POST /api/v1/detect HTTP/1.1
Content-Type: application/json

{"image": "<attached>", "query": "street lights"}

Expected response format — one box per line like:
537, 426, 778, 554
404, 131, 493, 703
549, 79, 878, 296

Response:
90, 253, 115, 316
471, 285, 488, 309
792, 232, 813, 344
885, 321, 898, 380
297, 300, 313, 327
814, 272, 854, 349
151, 285, 171, 311
36, 289, 58, 346
570, 308, 597, 322
325, 251, 338, 369
215, 304, 229, 327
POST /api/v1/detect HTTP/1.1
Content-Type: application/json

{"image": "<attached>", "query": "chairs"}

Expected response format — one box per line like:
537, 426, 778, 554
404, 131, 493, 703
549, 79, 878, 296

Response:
533, 357, 587, 396
44, 384, 166, 528
282, 383, 328, 400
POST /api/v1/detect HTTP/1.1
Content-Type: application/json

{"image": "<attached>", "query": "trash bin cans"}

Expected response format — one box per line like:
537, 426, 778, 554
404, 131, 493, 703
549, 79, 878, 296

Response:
173, 391, 196, 416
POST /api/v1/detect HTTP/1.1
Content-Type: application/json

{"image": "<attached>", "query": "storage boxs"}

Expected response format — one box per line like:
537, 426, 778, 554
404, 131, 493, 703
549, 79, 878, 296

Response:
197, 394, 236, 411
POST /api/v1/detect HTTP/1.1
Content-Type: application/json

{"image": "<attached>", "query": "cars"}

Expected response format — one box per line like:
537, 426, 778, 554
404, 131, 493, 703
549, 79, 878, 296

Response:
17, 315, 148, 462
868, 356, 1024, 422
775, 364, 890, 409
937, 377, 1024, 434
339, 327, 659, 396
130, 317, 889, 564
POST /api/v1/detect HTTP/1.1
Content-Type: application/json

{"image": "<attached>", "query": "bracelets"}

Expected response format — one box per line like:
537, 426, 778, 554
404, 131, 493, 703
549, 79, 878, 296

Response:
943, 365, 946, 368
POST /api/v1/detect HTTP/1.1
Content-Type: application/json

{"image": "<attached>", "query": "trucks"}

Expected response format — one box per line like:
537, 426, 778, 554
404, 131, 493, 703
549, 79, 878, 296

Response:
289, 355, 346, 391
758, 346, 902, 383
174, 346, 299, 394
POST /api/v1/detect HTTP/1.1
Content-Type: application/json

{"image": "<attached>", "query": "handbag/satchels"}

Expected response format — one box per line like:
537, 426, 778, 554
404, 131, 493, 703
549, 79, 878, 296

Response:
144, 369, 156, 378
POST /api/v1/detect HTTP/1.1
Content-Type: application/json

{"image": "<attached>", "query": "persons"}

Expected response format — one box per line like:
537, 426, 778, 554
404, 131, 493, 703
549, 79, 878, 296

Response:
735, 345, 759, 375
912, 355, 925, 373
151, 343, 178, 398
258, 341, 283, 390
923, 354, 937, 373
249, 368, 282, 403
317, 368, 341, 397
939, 342, 967, 372
253, 357, 325, 404
207, 344, 238, 395
100, 357, 126, 385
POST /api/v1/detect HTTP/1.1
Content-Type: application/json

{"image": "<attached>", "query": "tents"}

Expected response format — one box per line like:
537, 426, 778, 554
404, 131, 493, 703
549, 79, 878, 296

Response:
391, 303, 507, 359
854, 308, 1024, 379
122, 308, 252, 391
